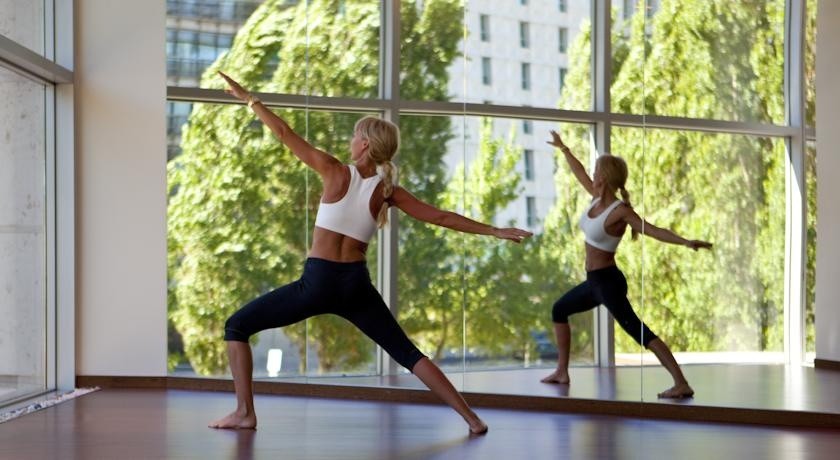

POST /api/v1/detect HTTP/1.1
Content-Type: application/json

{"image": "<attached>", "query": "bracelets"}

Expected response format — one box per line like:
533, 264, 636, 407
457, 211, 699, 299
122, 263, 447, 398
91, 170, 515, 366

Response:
247, 96, 261, 108
560, 146, 569, 154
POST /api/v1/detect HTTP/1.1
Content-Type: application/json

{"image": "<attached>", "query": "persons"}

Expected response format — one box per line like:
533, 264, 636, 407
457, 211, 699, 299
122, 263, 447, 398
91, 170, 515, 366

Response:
539, 129, 714, 398
207, 69, 534, 434
